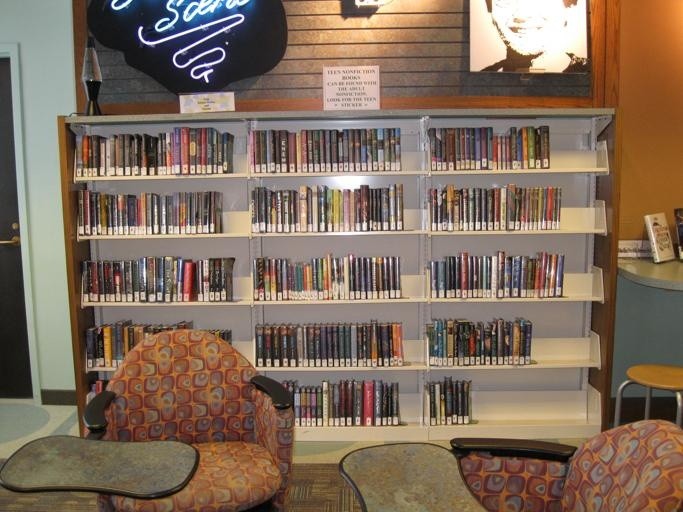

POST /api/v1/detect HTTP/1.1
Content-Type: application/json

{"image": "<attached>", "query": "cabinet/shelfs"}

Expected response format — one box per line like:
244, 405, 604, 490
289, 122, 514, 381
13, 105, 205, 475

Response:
56, 95, 619, 445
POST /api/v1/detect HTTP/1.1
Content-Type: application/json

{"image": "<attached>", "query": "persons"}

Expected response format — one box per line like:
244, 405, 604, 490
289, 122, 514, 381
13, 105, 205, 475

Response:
479, 0, 589, 73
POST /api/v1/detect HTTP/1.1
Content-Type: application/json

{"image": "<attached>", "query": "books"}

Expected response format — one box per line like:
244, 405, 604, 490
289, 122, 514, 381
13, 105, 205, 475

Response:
246, 130, 405, 232
644, 211, 676, 264
85, 319, 232, 368
673, 207, 683, 263
253, 258, 403, 427
82, 256, 234, 303
74, 123, 233, 176
429, 250, 566, 366
78, 190, 221, 235
428, 127, 562, 231
93, 380, 109, 395
429, 376, 472, 425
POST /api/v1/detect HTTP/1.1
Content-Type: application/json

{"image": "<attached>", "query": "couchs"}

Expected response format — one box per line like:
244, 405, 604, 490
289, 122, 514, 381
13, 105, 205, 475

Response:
338, 418, 683, 512
84, 328, 297, 511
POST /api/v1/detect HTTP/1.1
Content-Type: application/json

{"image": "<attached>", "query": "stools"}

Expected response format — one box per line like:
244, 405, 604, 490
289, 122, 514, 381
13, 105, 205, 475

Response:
613, 363, 682, 430
